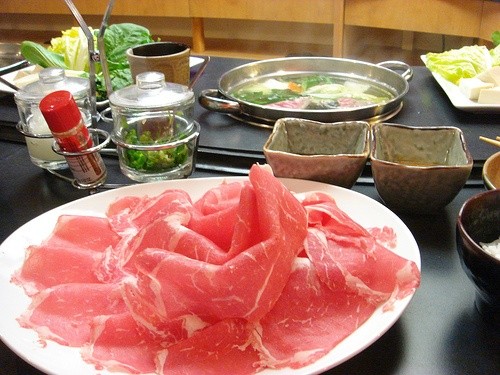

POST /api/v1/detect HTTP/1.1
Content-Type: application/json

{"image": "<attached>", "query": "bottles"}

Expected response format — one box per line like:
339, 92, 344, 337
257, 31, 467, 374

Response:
40, 92, 108, 187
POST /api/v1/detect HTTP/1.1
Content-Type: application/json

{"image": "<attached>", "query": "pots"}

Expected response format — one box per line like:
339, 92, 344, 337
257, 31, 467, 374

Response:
199, 56, 413, 122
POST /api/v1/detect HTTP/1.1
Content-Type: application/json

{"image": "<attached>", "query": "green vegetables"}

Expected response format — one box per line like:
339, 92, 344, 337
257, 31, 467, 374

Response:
94, 21, 153, 98
236, 75, 332, 104
20, 39, 66, 69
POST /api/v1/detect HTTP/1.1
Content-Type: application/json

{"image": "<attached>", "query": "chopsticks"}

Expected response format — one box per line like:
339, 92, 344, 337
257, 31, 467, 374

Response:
479, 136, 500, 147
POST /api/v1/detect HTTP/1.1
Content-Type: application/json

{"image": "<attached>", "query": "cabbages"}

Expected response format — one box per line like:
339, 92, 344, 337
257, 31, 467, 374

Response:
425, 44, 500, 84
50, 22, 100, 72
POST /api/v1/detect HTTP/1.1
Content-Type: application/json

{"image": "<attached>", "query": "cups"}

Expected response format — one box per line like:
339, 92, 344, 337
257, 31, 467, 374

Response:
14, 67, 95, 170
126, 42, 191, 88
108, 72, 195, 182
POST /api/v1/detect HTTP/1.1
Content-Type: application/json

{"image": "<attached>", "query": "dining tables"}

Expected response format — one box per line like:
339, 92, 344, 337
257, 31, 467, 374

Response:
0, 55, 500, 375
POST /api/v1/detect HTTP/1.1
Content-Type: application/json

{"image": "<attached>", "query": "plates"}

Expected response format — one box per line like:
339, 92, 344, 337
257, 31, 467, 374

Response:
420, 55, 500, 112
0, 174, 421, 375
0, 57, 205, 93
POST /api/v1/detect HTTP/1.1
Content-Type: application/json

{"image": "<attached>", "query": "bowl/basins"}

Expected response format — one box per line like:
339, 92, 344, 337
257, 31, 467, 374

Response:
368, 123, 473, 212
263, 117, 371, 188
482, 151, 500, 191
455, 189, 500, 315
